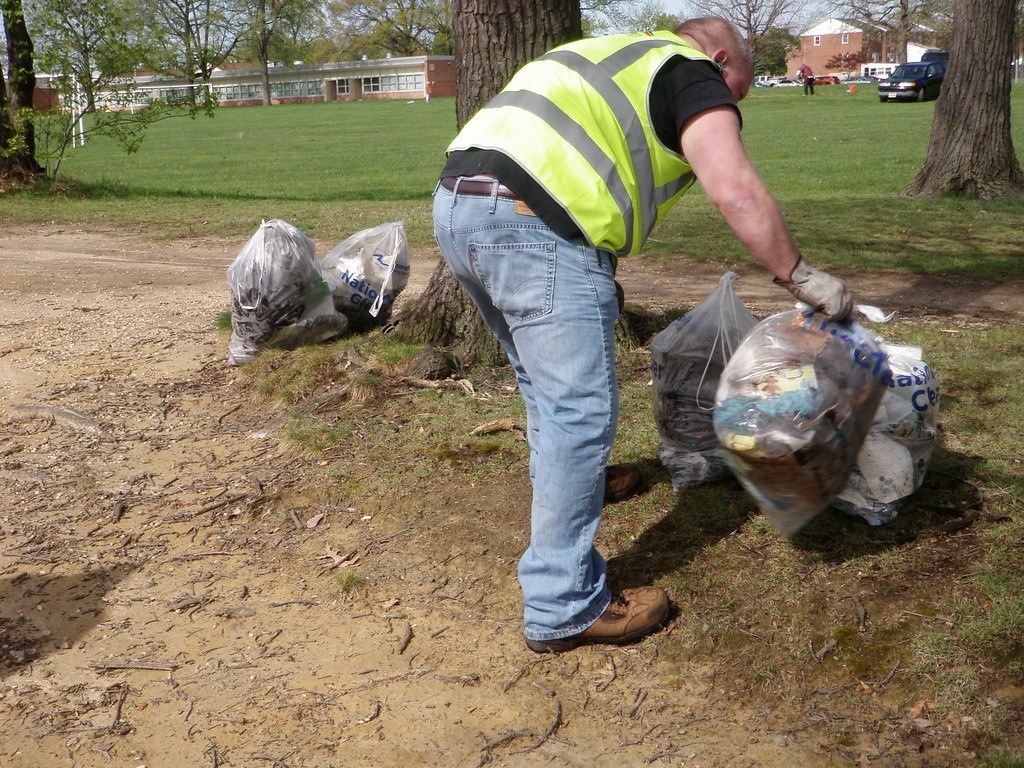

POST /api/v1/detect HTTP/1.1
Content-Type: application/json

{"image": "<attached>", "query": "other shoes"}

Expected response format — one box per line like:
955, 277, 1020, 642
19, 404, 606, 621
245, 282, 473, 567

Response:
523, 586, 669, 653
602, 463, 644, 509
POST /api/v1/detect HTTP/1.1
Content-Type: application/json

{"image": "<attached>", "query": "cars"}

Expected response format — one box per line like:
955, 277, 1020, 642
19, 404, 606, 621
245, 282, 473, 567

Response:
920, 48, 950, 63
878, 60, 948, 104
754, 75, 879, 88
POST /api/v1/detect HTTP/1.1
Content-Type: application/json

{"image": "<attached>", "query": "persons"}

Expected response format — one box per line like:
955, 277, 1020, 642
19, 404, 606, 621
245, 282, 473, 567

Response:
432, 16, 854, 654
796, 64, 815, 97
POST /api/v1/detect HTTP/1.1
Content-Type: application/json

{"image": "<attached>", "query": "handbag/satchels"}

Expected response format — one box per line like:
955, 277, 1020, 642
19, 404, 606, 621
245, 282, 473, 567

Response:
795, 302, 940, 526
651, 272, 760, 495
226, 219, 349, 368
320, 219, 410, 330
712, 310, 893, 540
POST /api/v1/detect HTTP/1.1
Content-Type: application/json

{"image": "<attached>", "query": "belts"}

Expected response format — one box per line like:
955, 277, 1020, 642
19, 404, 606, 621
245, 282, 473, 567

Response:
441, 176, 522, 200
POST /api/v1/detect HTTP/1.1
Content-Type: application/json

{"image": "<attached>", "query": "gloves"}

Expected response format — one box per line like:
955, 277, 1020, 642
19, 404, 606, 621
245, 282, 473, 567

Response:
773, 254, 853, 323
